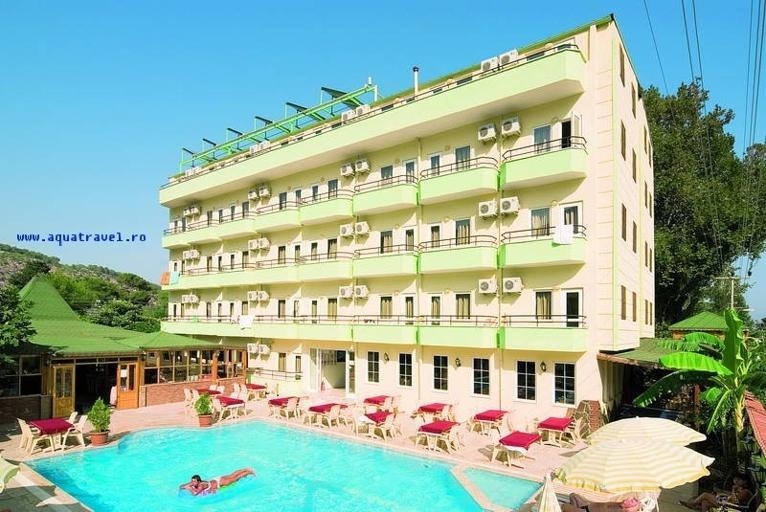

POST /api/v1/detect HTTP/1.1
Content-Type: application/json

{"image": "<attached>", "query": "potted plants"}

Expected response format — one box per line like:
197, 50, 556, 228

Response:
195, 391, 215, 427
87, 396, 112, 447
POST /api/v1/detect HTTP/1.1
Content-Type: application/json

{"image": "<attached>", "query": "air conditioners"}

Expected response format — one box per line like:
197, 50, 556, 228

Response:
340, 224, 354, 240
339, 284, 353, 301
189, 294, 200, 305
182, 250, 191, 260
257, 290, 270, 302
340, 162, 355, 178
188, 250, 200, 259
258, 237, 271, 252
356, 104, 371, 117
183, 206, 192, 218
356, 157, 371, 175
247, 291, 258, 302
478, 278, 498, 296
250, 145, 259, 153
258, 343, 272, 358
191, 204, 201, 215
498, 48, 518, 67
259, 185, 272, 198
247, 187, 259, 202
476, 121, 497, 146
352, 284, 369, 301
259, 140, 270, 149
499, 195, 520, 217
481, 56, 498, 72
340, 109, 354, 123
181, 294, 191, 305
248, 343, 259, 355
354, 220, 370, 238
248, 240, 260, 252
478, 199, 497, 221
503, 277, 524, 297
501, 116, 522, 137
185, 166, 203, 176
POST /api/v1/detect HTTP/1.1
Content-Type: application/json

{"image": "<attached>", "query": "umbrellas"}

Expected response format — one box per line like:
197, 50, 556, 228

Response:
588, 415, 709, 447
555, 437, 711, 494
539, 472, 562, 512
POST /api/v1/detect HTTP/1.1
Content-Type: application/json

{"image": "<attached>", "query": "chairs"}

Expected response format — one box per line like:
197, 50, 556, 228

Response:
713, 480, 764, 512
15, 408, 87, 451
553, 484, 660, 511
306, 390, 586, 469
184, 382, 303, 423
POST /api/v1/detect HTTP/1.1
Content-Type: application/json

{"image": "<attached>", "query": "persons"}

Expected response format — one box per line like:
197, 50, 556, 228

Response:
680, 473, 751, 511
178, 467, 256, 496
